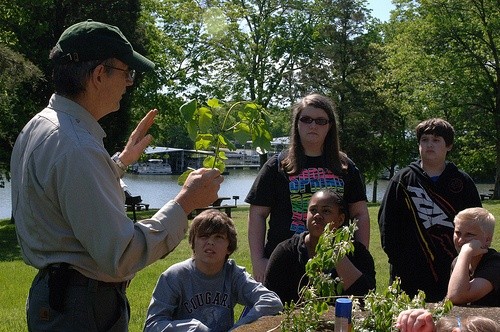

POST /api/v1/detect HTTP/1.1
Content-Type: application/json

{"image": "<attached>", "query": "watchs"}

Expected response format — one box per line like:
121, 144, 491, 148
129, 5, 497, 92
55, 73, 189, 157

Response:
110, 152, 128, 170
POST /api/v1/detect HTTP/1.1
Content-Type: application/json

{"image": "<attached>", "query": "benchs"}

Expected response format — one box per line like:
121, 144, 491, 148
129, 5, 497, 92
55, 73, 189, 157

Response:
124, 203, 149, 211
187, 206, 237, 220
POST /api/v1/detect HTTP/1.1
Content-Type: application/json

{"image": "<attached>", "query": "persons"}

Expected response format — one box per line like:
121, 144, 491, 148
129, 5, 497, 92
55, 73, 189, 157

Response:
263, 188, 377, 307
143, 209, 283, 332
244, 93, 370, 283
377, 118, 482, 303
11, 19, 224, 332
446, 207, 500, 307
396, 309, 500, 332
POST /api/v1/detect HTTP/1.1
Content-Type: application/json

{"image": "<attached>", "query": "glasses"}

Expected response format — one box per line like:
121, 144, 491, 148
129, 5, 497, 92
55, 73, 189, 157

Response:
299, 115, 331, 125
105, 65, 136, 79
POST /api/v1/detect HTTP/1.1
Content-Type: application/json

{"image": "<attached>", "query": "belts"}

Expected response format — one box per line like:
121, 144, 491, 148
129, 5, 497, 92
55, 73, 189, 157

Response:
38, 268, 122, 287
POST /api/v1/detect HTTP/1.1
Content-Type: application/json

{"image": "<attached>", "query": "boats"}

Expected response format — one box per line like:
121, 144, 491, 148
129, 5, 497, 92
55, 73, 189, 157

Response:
377, 165, 399, 179
135, 158, 172, 175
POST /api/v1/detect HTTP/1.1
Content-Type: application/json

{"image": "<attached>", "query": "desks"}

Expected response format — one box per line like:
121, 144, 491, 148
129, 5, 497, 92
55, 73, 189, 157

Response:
232, 301, 500, 332
212, 198, 230, 206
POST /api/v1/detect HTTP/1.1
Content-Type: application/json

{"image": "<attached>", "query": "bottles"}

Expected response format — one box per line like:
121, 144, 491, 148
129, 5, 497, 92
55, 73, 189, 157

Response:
333, 297, 353, 332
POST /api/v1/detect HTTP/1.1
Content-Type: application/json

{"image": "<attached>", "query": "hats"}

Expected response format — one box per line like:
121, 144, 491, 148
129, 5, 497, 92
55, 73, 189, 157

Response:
49, 18, 155, 71
